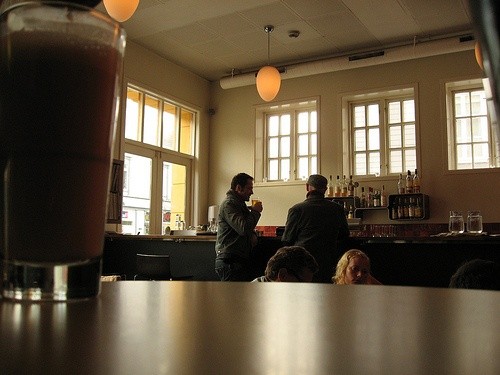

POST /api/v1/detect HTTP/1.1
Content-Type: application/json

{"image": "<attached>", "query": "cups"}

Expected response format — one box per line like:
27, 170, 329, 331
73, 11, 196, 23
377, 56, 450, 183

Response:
0, 1, 127, 302
251, 197, 259, 208
448, 210, 465, 233
467, 209, 483, 233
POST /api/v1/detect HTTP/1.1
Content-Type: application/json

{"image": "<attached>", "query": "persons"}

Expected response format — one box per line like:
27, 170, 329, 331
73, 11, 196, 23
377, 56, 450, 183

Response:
331, 249, 382, 286
281, 175, 350, 283
251, 246, 318, 283
214, 173, 263, 281
449, 259, 500, 291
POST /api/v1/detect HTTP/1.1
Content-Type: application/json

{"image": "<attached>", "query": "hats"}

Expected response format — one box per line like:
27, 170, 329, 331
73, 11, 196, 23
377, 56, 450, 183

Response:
308, 175, 327, 186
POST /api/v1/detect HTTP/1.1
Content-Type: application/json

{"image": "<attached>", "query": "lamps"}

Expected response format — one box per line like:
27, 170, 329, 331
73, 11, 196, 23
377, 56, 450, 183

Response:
103, 0, 139, 23
256, 24, 282, 102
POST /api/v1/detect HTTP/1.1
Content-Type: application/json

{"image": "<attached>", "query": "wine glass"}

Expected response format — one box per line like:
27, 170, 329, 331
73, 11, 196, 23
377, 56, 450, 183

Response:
362, 225, 397, 238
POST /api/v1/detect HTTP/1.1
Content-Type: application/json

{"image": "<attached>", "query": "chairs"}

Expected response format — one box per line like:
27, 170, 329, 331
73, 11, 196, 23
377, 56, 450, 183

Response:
132, 254, 194, 282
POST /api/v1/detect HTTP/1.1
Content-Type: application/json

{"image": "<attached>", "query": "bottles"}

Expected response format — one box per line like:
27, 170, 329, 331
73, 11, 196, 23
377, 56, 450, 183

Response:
405, 170, 413, 193
391, 197, 422, 219
413, 169, 421, 193
397, 173, 405, 194
326, 174, 387, 220
